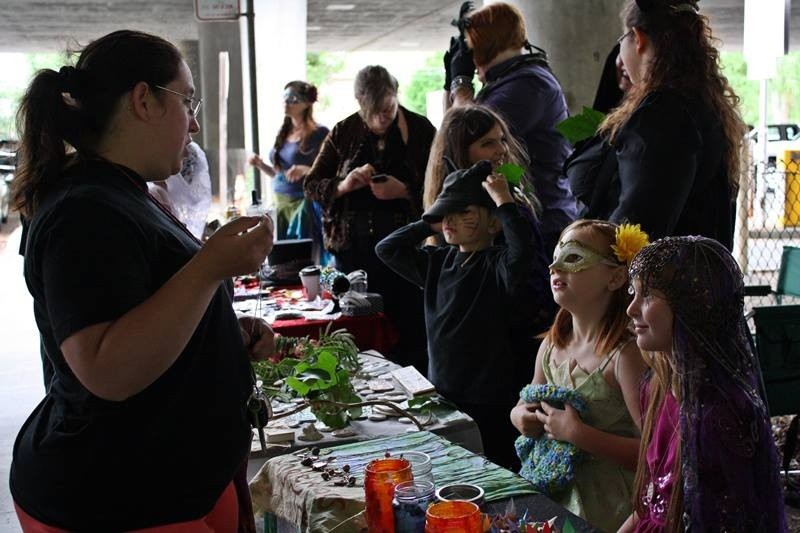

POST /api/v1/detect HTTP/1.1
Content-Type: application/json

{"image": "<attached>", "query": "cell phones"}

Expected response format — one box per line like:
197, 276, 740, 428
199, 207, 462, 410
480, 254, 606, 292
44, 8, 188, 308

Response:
371, 174, 388, 183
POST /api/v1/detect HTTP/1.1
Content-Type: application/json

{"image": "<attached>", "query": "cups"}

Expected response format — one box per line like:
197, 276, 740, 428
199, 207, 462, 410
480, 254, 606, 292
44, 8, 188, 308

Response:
246, 207, 260, 232
299, 266, 321, 300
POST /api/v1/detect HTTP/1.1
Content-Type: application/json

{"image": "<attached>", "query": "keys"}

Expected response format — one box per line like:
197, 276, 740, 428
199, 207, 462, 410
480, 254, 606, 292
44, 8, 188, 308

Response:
245, 399, 268, 453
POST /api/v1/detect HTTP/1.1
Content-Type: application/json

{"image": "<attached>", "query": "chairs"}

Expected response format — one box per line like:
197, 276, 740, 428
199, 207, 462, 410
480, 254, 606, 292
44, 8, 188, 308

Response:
744, 245, 800, 415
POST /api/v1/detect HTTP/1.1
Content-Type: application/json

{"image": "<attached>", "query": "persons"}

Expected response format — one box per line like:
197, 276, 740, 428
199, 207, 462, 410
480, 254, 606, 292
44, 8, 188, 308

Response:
302, 65, 436, 367
562, 0, 741, 252
10, 30, 273, 533
250, 82, 330, 268
510, 220, 649, 533
424, 3, 577, 231
374, 160, 551, 466
618, 235, 787, 533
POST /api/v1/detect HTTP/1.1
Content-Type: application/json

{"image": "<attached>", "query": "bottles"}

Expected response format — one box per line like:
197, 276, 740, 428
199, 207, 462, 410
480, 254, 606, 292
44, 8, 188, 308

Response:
364, 458, 414, 533
436, 484, 486, 533
393, 480, 435, 533
425, 501, 482, 533
390, 451, 435, 485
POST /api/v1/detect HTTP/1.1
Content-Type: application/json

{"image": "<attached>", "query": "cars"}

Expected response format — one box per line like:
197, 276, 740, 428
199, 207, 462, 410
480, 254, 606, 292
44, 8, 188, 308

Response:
0, 139, 19, 179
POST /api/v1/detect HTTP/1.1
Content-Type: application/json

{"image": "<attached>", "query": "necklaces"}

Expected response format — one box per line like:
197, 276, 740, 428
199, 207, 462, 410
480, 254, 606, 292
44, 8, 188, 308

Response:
647, 419, 679, 503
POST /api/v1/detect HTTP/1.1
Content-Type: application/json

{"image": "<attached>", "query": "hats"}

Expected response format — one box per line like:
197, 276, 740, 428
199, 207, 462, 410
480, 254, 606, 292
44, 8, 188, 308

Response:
421, 159, 515, 223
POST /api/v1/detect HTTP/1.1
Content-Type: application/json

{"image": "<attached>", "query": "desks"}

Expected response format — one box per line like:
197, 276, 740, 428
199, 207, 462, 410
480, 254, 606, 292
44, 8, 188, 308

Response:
249, 431, 607, 533
268, 285, 400, 353
246, 350, 484, 533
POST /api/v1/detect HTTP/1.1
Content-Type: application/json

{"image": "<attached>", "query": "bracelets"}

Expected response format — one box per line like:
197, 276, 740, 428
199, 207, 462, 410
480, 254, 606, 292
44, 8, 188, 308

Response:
450, 76, 473, 90
449, 86, 474, 104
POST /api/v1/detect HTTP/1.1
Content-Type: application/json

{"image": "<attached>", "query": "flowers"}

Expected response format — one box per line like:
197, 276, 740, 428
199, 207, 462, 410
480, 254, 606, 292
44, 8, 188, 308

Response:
610, 222, 651, 268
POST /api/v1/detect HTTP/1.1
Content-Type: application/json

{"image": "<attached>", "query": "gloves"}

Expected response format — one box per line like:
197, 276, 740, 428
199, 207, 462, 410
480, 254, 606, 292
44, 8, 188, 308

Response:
443, 37, 470, 89
450, 35, 476, 80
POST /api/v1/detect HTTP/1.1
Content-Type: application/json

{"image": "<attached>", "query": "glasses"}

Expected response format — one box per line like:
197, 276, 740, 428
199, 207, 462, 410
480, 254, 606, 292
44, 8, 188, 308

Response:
152, 84, 203, 118
617, 31, 634, 44
